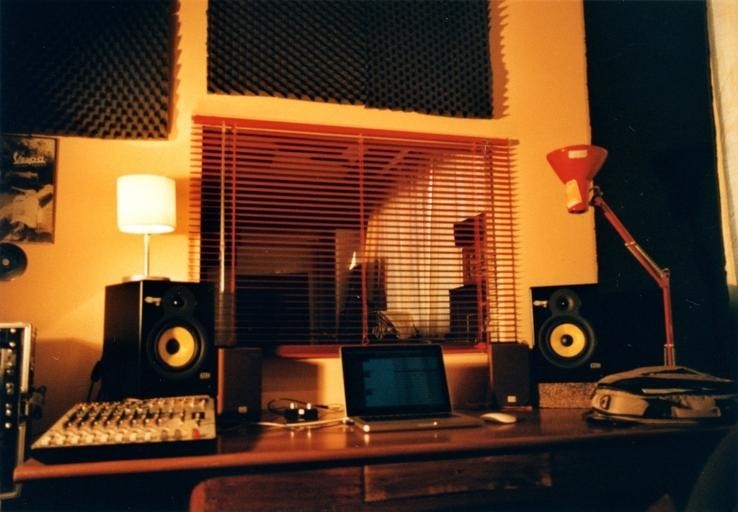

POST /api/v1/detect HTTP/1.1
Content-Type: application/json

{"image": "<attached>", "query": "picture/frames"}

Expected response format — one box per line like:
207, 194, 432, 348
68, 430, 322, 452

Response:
3, 133, 60, 246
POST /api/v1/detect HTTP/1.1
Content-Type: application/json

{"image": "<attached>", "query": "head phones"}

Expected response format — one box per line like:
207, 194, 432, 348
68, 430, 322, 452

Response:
267, 398, 328, 422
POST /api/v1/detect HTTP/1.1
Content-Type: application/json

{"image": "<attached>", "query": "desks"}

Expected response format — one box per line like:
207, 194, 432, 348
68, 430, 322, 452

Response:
12, 403, 737, 512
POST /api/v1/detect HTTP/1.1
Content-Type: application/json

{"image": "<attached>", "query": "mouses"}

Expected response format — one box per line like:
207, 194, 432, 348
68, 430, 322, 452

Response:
480, 412, 516, 423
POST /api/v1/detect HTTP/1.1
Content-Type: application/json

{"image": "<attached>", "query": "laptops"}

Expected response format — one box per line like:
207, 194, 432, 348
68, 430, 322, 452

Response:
339, 344, 485, 433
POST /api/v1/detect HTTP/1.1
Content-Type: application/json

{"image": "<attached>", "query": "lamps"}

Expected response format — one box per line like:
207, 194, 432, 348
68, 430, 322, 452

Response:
119, 174, 176, 278
546, 144, 727, 384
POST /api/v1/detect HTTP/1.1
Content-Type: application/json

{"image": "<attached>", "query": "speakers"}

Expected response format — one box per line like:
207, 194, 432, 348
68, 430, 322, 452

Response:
99, 280, 217, 417
529, 284, 607, 383
216, 347, 263, 424
486, 342, 533, 411
